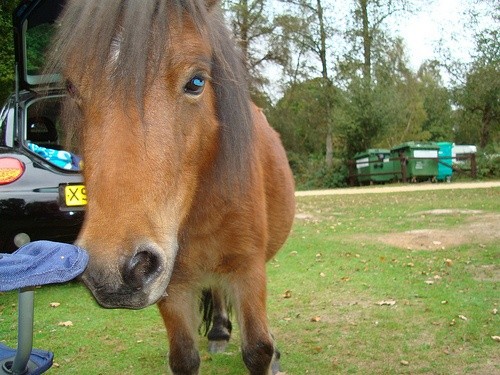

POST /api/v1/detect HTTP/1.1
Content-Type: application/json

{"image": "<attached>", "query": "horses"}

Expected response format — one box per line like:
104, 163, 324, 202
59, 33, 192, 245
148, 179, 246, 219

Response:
38, 1, 297, 375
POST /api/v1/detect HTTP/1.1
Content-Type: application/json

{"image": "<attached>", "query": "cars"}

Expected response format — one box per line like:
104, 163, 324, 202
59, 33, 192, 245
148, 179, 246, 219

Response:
0, 2, 85, 235
